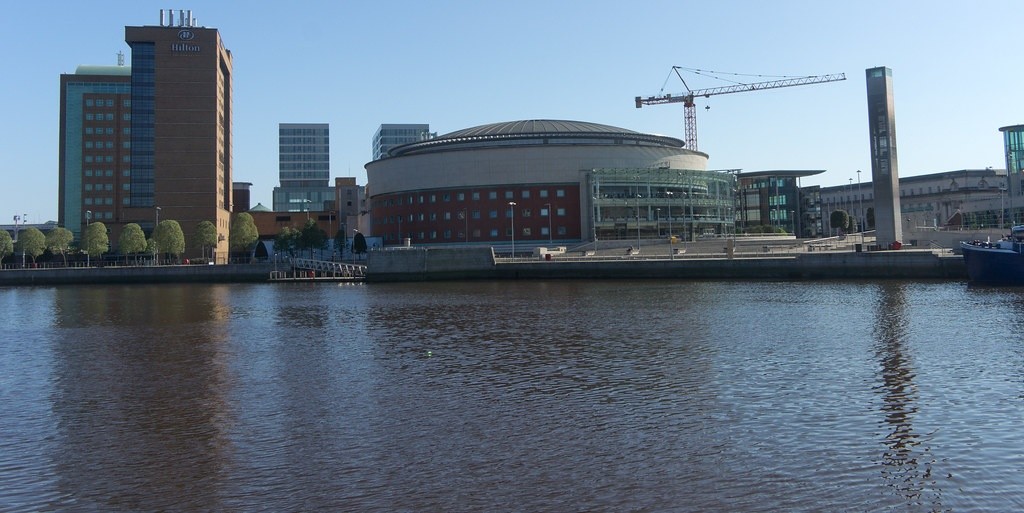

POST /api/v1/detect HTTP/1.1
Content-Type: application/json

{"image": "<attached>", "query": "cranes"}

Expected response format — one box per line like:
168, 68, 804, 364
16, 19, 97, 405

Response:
635, 66, 847, 150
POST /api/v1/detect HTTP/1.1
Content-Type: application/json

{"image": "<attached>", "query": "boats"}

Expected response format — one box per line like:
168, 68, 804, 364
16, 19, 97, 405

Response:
959, 225, 1024, 288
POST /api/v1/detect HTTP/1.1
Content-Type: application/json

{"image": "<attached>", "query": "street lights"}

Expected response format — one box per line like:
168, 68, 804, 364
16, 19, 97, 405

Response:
508, 202, 516, 262
656, 208, 660, 238
544, 203, 552, 244
22, 214, 27, 269
155, 206, 162, 227
999, 188, 1006, 229
13, 215, 20, 241
666, 192, 674, 259
86, 211, 91, 266
636, 194, 641, 249
307, 200, 311, 221
464, 208, 467, 245
849, 178, 854, 234
329, 211, 335, 234
592, 197, 597, 254
857, 170, 864, 232
